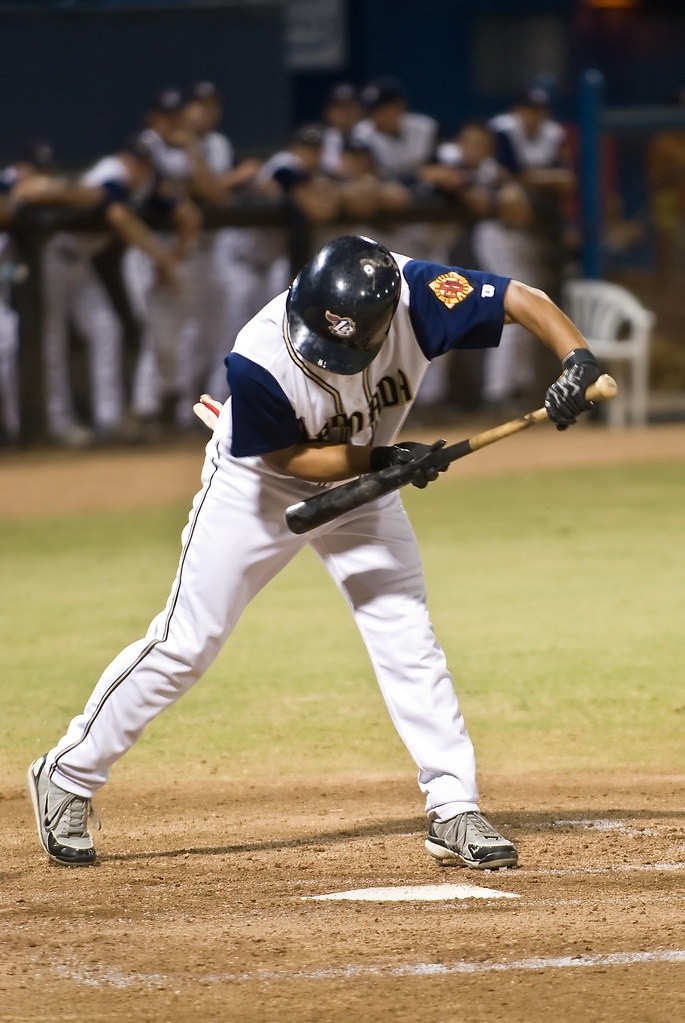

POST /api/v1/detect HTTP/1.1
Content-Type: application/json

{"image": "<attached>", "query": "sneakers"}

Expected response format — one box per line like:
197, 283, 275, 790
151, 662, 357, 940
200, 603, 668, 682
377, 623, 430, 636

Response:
27, 752, 96, 866
425, 812, 518, 869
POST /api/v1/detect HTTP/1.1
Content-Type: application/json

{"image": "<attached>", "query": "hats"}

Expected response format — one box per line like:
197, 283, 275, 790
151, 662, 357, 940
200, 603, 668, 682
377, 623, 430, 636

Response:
185, 81, 223, 104
519, 87, 554, 114
155, 90, 184, 114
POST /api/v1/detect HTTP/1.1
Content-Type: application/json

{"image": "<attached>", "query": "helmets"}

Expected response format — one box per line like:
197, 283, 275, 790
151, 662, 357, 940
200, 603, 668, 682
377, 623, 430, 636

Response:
285, 234, 401, 375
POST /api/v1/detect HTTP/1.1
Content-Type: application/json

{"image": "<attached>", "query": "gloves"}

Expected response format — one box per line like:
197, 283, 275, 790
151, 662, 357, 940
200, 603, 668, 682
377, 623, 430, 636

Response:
544, 348, 601, 431
371, 440, 449, 489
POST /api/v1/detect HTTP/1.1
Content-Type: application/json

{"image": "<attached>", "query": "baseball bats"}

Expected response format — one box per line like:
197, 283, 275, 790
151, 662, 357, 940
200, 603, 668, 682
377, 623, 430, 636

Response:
284, 369, 621, 534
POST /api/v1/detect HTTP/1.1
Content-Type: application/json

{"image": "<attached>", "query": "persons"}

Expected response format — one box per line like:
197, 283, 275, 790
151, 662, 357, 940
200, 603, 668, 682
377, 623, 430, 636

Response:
27, 233, 603, 869
0, 74, 577, 452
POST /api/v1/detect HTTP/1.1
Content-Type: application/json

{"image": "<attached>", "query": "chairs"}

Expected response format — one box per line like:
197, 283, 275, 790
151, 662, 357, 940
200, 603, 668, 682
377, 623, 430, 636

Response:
556, 277, 656, 429
